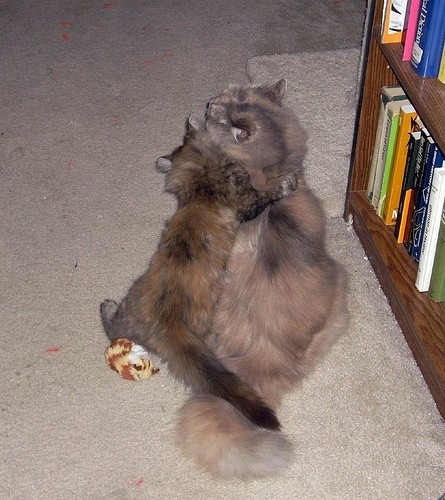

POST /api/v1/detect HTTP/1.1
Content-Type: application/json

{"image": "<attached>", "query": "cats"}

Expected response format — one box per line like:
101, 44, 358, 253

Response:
173, 78, 351, 481
99, 115, 300, 432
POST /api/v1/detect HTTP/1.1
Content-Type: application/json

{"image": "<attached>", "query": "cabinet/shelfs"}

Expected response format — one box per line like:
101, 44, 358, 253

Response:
343, 0, 445, 424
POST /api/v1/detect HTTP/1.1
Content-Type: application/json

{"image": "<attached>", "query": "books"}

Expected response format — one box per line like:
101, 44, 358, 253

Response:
366, 0, 444, 304
